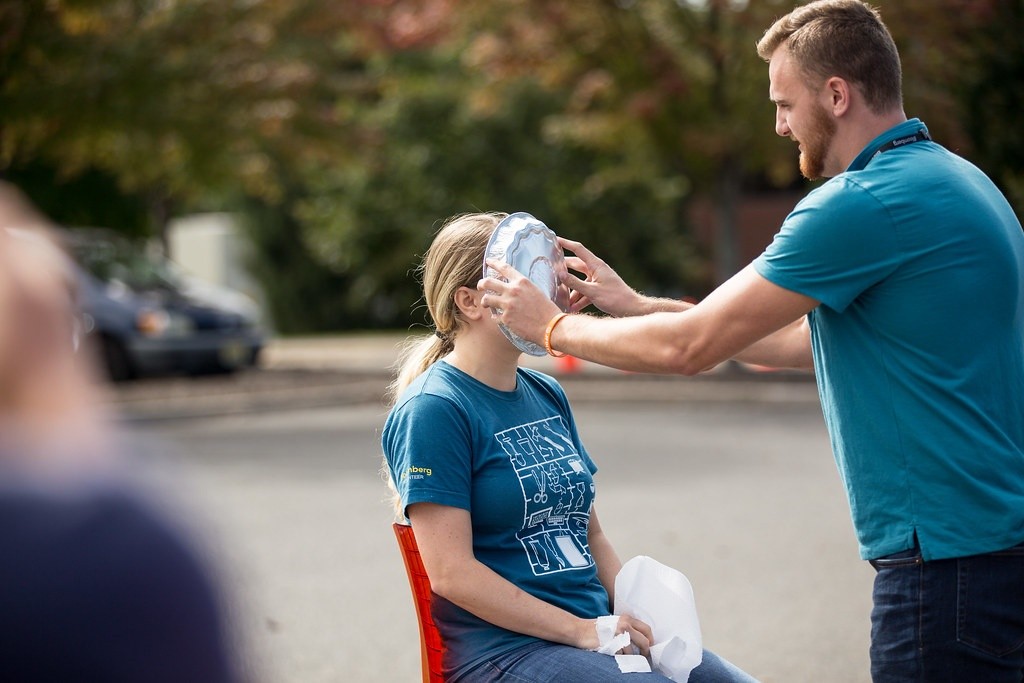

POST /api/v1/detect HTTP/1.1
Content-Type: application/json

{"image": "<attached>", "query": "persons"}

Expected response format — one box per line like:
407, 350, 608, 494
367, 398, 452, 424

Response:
0, 179, 239, 683
381, 212, 765, 683
477, 0, 1024, 683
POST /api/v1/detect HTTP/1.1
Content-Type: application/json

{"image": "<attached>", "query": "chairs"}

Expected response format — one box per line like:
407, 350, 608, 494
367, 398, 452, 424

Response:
393, 522, 445, 683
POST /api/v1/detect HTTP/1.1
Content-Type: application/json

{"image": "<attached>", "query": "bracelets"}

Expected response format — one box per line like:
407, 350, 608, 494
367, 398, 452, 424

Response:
543, 314, 568, 357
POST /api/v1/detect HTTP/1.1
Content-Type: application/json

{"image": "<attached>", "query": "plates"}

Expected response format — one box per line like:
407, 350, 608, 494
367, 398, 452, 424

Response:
483, 211, 570, 356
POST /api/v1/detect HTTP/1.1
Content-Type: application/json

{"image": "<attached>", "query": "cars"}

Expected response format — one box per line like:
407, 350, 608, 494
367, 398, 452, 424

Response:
58, 226, 265, 383
